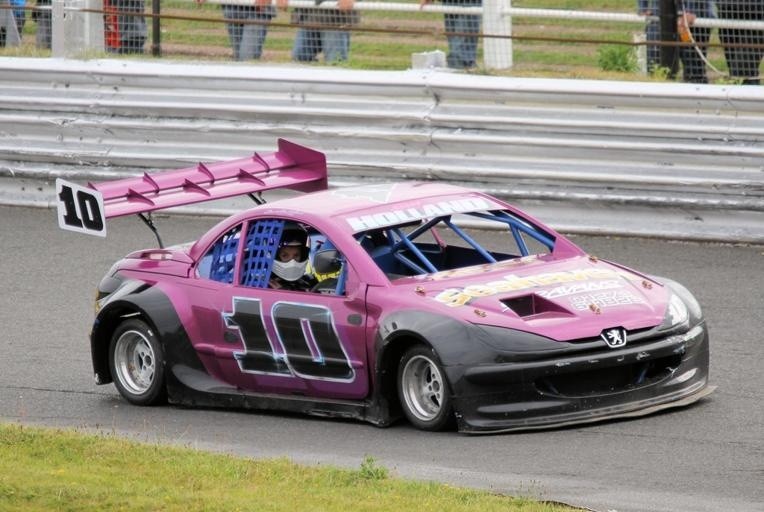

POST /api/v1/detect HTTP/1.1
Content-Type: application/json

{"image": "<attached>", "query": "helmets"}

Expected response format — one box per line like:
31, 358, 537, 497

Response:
264, 221, 310, 281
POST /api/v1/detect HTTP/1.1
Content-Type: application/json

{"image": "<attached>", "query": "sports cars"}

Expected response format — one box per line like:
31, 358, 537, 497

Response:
51, 136, 719, 436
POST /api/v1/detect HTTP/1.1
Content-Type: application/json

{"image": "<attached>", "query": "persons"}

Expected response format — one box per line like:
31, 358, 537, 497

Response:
419, 0, 482, 69
196, 0, 276, 61
636, 0, 714, 82
258, 220, 322, 293
0, 0, 147, 55
276, 0, 359, 64
715, 0, 763, 84
